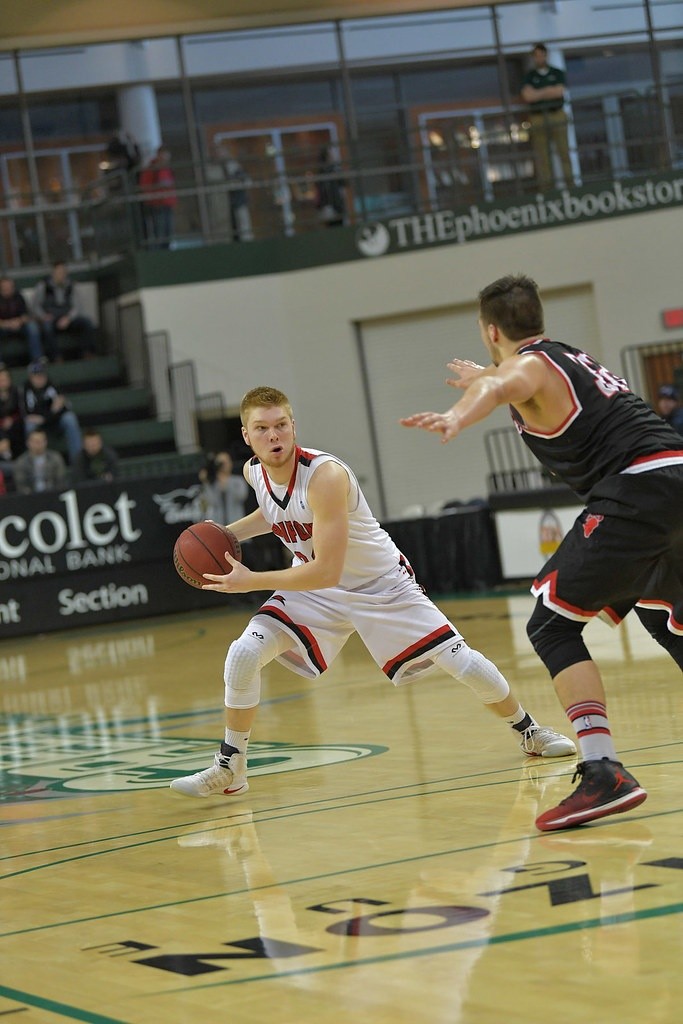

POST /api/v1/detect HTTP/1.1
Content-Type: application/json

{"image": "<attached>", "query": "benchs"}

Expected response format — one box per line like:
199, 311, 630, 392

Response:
0, 271, 229, 499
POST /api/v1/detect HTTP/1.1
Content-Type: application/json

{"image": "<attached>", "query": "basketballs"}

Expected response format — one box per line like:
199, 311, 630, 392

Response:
172, 521, 244, 591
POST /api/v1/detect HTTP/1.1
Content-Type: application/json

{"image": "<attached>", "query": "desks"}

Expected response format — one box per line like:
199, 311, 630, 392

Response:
485, 482, 598, 592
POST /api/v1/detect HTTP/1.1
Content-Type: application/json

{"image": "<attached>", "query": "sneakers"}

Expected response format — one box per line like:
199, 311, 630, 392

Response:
512, 713, 577, 757
533, 822, 654, 876
520, 757, 577, 798
171, 741, 249, 798
535, 756, 647, 831
178, 809, 259, 860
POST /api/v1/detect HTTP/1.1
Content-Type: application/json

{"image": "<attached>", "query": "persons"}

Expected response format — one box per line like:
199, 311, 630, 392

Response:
519, 40, 581, 202
399, 271, 683, 832
170, 386, 578, 798
0, 133, 352, 612
